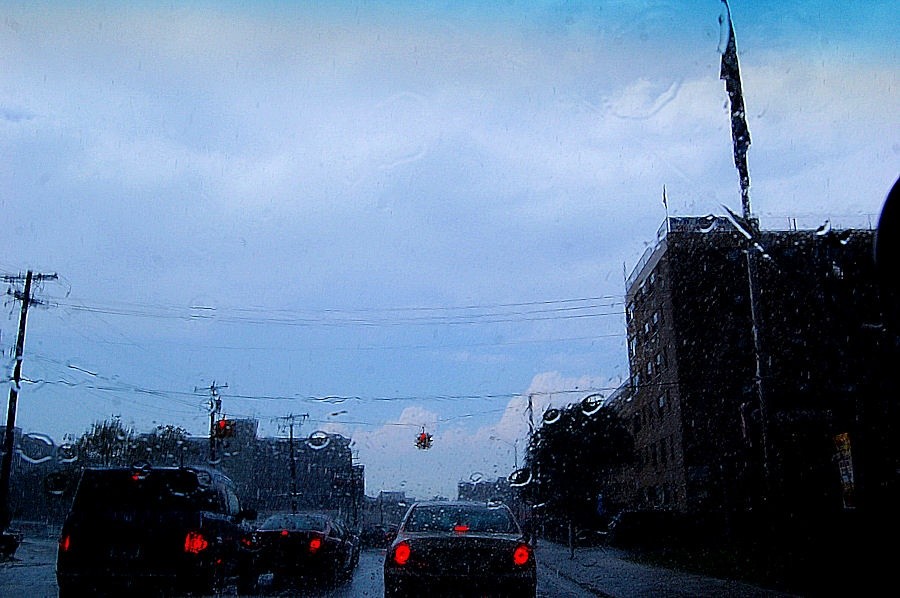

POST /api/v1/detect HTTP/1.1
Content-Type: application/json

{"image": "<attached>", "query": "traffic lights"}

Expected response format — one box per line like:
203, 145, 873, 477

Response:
216, 419, 237, 439
413, 432, 433, 450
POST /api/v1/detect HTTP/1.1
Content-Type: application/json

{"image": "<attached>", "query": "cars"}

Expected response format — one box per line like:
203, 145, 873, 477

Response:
373, 498, 540, 598
239, 511, 361, 596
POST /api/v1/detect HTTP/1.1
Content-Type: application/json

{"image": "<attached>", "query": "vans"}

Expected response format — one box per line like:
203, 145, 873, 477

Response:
53, 459, 260, 598
